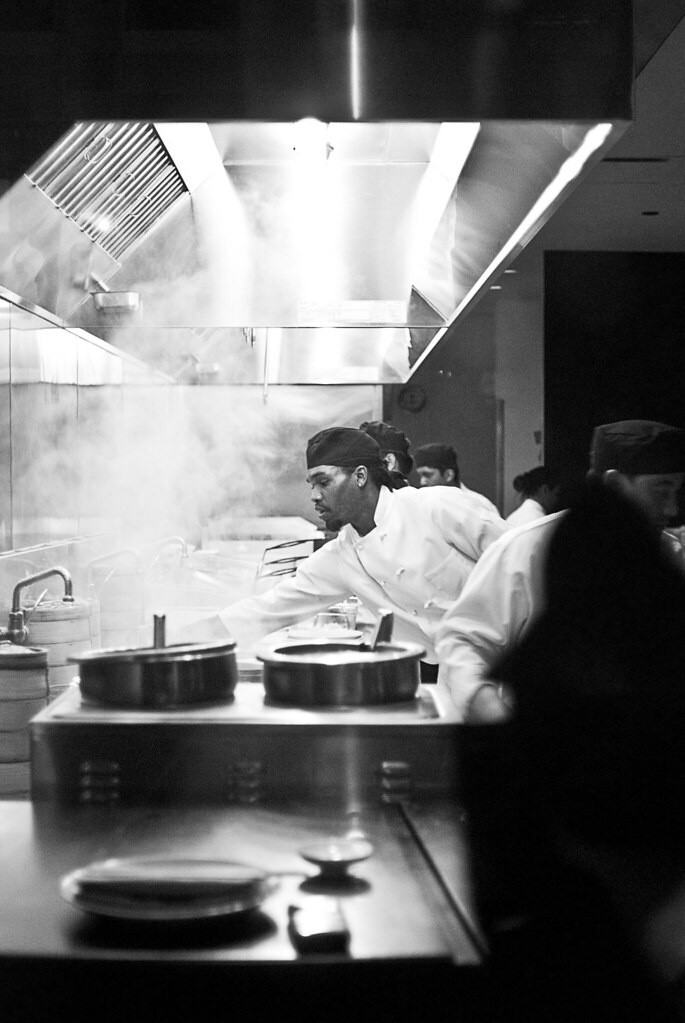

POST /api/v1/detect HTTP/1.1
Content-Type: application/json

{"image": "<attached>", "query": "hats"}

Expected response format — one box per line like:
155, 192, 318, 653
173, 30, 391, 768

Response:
361, 420, 410, 456
307, 426, 384, 468
415, 444, 456, 470
590, 419, 685, 474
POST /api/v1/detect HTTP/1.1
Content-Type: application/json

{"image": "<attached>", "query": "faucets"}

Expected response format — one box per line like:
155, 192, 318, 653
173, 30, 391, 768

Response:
0, 566, 75, 649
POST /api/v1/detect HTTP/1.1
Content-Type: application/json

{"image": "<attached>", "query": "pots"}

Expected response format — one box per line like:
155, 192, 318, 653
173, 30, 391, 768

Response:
68, 643, 239, 709
256, 642, 427, 706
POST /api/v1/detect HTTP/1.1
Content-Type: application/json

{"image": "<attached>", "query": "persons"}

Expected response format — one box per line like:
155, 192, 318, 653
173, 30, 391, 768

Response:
415, 442, 504, 522
434, 418, 684, 726
505, 465, 572, 531
216, 425, 505, 665
465, 493, 684, 1023
358, 420, 415, 488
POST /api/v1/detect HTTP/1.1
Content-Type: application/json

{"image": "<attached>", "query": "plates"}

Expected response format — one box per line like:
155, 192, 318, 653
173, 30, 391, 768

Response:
298, 842, 373, 880
58, 855, 282, 919
